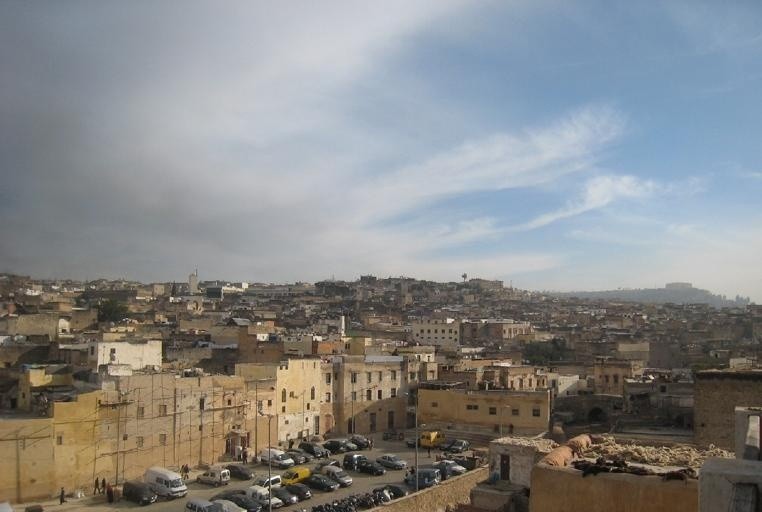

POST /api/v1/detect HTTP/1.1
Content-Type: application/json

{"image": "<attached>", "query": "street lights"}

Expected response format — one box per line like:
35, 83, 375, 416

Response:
351, 384, 379, 432
197, 402, 249, 462
255, 379, 267, 458
500, 405, 510, 439
402, 390, 419, 492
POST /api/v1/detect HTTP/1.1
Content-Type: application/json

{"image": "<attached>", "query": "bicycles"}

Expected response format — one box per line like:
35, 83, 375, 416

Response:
312, 492, 386, 512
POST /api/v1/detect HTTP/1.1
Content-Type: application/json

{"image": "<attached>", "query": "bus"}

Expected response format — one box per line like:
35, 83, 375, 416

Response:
420, 430, 445, 448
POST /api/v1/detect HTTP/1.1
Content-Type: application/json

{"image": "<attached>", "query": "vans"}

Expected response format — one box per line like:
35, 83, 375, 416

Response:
261, 447, 295, 469
123, 480, 158, 505
143, 466, 187, 500
321, 465, 353, 486
404, 469, 441, 485
343, 453, 366, 470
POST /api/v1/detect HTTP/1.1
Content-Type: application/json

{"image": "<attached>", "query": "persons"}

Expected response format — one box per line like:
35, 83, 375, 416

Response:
304, 437, 308, 441
238, 447, 248, 465
404, 466, 415, 479
366, 437, 374, 451
289, 439, 294, 448
58, 487, 68, 506
179, 464, 190, 480
93, 477, 114, 504
427, 446, 442, 461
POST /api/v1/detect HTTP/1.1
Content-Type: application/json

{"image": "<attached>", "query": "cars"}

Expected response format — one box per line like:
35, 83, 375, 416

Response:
406, 436, 421, 449
361, 462, 386, 476
406, 436, 420, 447
310, 474, 340, 491
373, 485, 409, 498
289, 435, 332, 464
382, 429, 403, 441
185, 464, 312, 512
432, 439, 470, 475
325, 434, 372, 454
377, 453, 408, 469
